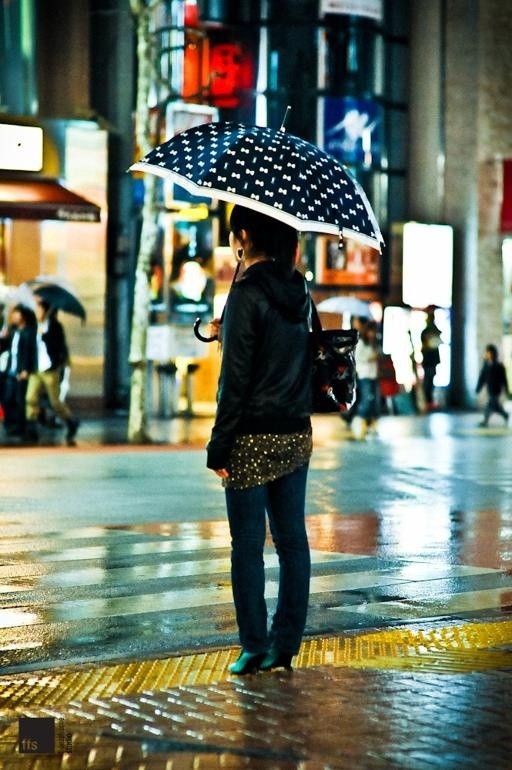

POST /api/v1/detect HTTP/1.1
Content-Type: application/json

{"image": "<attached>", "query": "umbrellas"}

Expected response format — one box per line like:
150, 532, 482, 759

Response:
316, 296, 375, 329
124, 106, 387, 344
34, 283, 88, 325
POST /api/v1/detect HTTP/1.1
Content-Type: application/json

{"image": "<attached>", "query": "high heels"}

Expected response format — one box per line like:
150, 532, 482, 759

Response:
228, 648, 267, 673
260, 642, 292, 669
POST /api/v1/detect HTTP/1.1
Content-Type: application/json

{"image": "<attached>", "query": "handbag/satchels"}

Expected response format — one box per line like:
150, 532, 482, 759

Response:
306, 329, 357, 414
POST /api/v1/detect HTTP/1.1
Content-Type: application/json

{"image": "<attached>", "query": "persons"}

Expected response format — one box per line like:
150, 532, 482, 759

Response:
203, 205, 323, 676
0, 299, 80, 447
352, 315, 381, 433
342, 320, 381, 440
474, 344, 512, 428
419, 311, 442, 407
